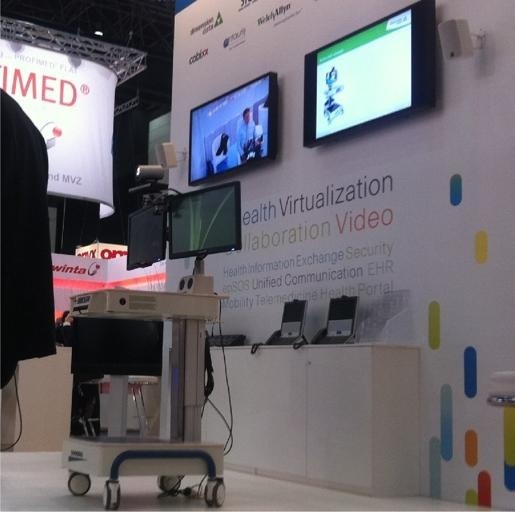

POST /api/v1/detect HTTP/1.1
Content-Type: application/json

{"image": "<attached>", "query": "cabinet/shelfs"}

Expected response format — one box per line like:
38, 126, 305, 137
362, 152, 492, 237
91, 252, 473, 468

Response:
202, 345, 421, 498
1, 343, 74, 452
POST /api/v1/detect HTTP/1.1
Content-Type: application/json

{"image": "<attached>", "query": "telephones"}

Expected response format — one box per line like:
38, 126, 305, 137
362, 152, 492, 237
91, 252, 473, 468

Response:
311, 295, 358, 345
267, 298, 308, 344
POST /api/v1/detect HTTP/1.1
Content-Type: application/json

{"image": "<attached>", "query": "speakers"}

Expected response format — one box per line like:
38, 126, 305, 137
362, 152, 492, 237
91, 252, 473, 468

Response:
438, 19, 474, 61
155, 142, 177, 168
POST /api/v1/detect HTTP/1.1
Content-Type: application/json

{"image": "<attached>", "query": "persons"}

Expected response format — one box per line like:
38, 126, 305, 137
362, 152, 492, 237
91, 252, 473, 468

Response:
236, 108, 262, 163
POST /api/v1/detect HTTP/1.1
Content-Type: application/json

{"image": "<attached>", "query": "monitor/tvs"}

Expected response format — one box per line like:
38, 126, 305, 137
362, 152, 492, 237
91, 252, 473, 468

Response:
127, 193, 167, 271
303, 1, 435, 148
168, 181, 242, 260
189, 71, 277, 186
